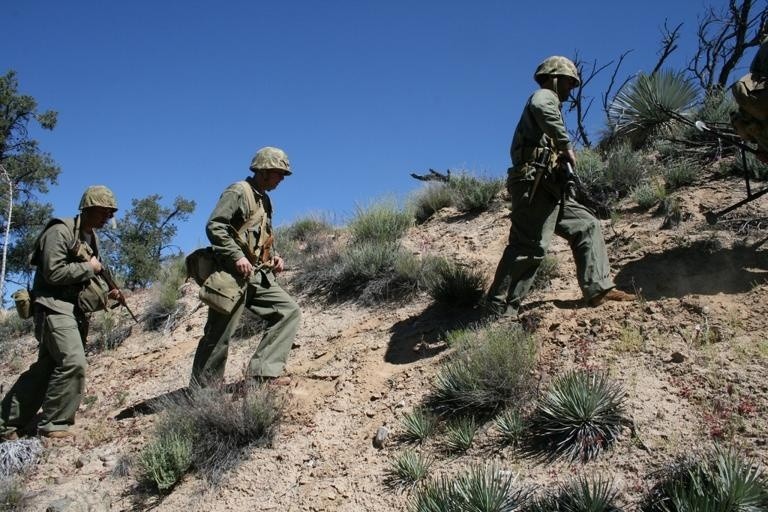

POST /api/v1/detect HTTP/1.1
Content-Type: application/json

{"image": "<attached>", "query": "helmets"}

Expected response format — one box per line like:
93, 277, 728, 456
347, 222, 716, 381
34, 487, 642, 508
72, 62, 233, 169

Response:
77, 185, 119, 214
248, 146, 293, 177
532, 55, 580, 89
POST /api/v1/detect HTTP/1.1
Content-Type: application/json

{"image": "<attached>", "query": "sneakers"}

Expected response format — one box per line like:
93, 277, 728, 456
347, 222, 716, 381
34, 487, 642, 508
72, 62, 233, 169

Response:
37, 429, 71, 439
1, 431, 20, 441
246, 376, 292, 387
591, 287, 637, 307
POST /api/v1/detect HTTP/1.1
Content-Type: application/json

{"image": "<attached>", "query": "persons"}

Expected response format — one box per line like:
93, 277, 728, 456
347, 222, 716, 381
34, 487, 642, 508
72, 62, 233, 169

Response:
184, 146, 301, 400
0, 186, 139, 441
470, 55, 636, 324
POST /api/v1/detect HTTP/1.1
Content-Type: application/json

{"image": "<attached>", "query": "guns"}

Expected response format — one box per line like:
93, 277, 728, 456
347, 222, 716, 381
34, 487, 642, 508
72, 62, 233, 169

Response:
69, 238, 136, 321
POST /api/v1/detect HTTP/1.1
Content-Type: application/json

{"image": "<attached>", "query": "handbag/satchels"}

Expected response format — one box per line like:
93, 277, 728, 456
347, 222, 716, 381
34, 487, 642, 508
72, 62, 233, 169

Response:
729, 71, 767, 155
185, 247, 219, 287
10, 290, 35, 319
77, 279, 109, 314
196, 271, 248, 316
